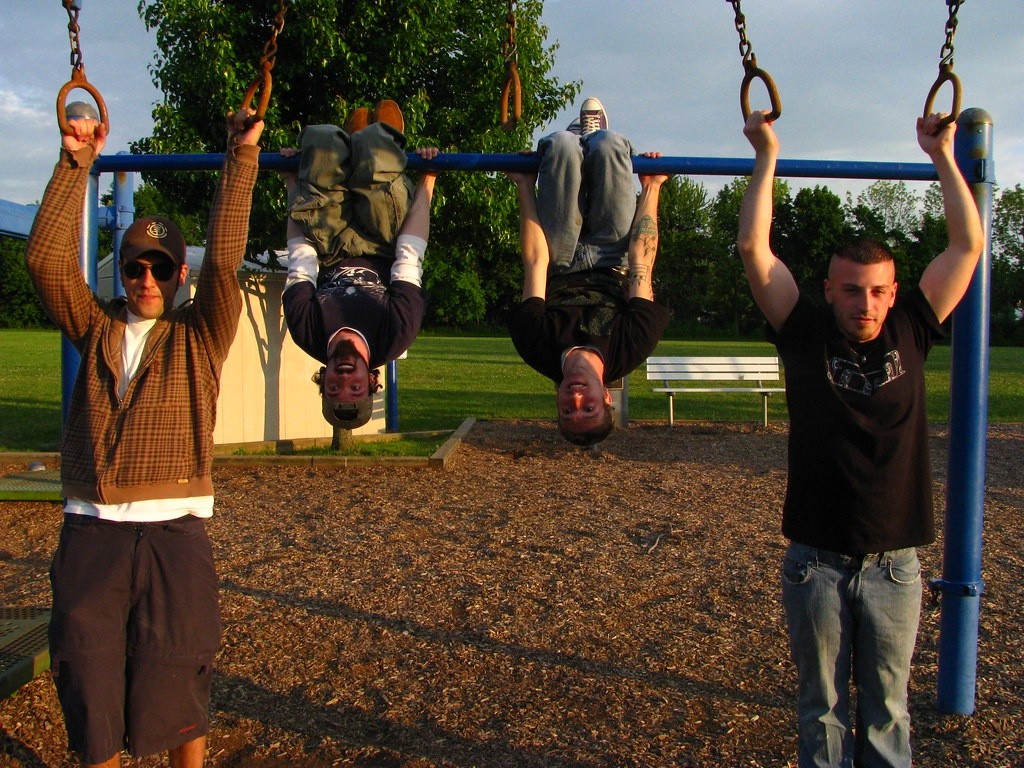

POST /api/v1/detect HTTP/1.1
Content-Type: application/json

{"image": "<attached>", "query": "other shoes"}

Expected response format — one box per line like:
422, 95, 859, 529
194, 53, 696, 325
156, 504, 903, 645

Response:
373, 99, 405, 133
345, 107, 373, 136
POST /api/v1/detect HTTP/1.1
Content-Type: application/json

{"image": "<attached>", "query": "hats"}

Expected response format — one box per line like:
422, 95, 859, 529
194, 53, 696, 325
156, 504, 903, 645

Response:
322, 396, 373, 430
120, 215, 186, 266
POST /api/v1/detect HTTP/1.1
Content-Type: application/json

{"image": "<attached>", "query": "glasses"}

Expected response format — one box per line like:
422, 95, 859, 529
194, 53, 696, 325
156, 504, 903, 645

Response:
124, 257, 176, 282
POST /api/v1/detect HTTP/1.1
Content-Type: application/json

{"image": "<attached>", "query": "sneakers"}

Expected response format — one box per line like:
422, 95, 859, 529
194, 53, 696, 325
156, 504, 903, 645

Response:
567, 118, 583, 141
580, 99, 607, 140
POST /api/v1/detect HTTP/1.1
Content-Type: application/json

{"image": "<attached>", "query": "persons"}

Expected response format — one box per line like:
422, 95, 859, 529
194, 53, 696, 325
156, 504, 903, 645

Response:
25, 108, 265, 768
280, 99, 439, 430
504, 98, 671, 446
737, 109, 985, 768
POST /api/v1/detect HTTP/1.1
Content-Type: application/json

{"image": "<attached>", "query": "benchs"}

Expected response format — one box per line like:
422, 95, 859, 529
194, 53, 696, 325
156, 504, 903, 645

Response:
647, 355, 786, 430
